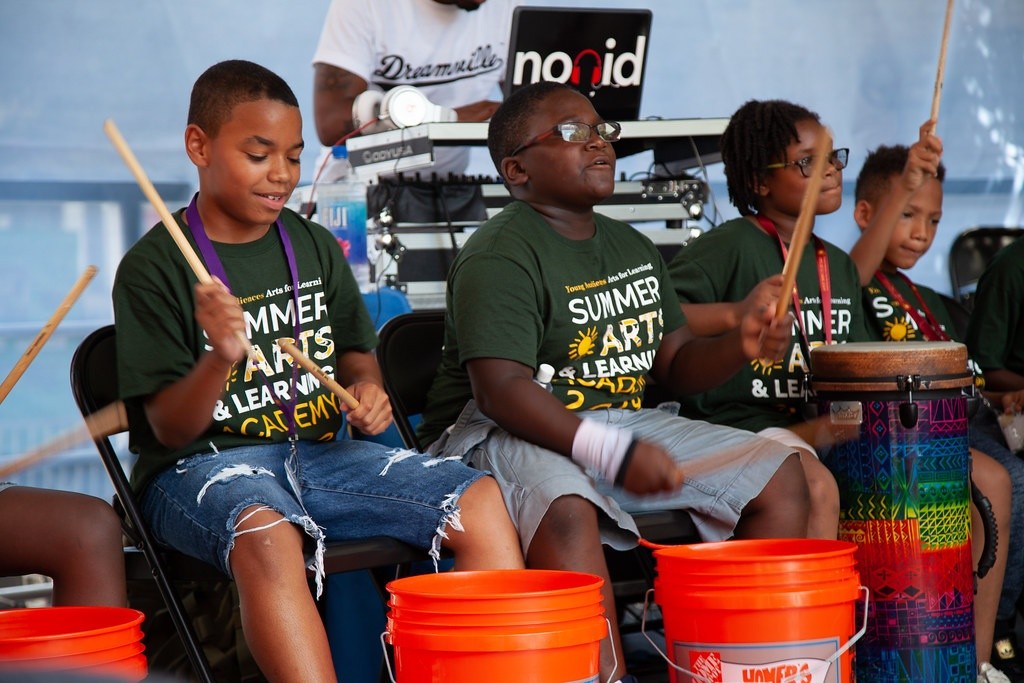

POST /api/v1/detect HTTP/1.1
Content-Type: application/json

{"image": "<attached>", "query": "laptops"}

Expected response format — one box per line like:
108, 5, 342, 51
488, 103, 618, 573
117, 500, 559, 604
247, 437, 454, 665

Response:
504, 6, 654, 121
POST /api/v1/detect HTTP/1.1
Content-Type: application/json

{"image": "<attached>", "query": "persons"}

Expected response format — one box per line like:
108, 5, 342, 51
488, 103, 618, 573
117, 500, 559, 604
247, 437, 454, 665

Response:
423, 82, 809, 683
112, 61, 527, 683
312, 0, 516, 282
0, 477, 127, 605
668, 101, 1024, 683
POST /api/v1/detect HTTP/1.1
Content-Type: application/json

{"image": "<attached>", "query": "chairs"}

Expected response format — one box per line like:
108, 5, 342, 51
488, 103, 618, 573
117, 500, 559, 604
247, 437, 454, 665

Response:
376, 310, 699, 640
69, 323, 454, 683
949, 226, 1024, 303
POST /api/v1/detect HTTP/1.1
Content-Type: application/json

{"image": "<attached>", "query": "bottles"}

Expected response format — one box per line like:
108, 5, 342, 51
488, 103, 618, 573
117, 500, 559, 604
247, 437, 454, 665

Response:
532, 363, 556, 394
315, 145, 372, 293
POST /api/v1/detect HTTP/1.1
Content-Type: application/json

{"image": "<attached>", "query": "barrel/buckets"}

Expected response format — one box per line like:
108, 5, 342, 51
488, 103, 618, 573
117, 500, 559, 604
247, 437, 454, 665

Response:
381, 569, 618, 683
0, 605, 150, 683
641, 537, 869, 683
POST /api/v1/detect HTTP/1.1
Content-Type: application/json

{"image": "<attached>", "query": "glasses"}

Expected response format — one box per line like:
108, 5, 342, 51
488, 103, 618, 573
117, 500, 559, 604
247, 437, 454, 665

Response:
758, 148, 849, 177
511, 120, 622, 157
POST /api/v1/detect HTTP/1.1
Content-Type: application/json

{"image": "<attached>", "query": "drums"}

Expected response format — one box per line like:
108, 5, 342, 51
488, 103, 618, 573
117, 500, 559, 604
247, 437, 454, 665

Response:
809, 340, 979, 683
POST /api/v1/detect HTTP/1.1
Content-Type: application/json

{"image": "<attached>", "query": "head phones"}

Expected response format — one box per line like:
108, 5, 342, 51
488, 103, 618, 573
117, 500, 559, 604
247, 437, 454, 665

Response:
350, 87, 459, 135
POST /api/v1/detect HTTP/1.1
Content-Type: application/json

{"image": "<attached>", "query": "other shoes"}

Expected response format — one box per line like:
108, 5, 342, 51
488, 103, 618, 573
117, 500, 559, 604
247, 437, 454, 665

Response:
977, 662, 1011, 683
614, 675, 638, 683
